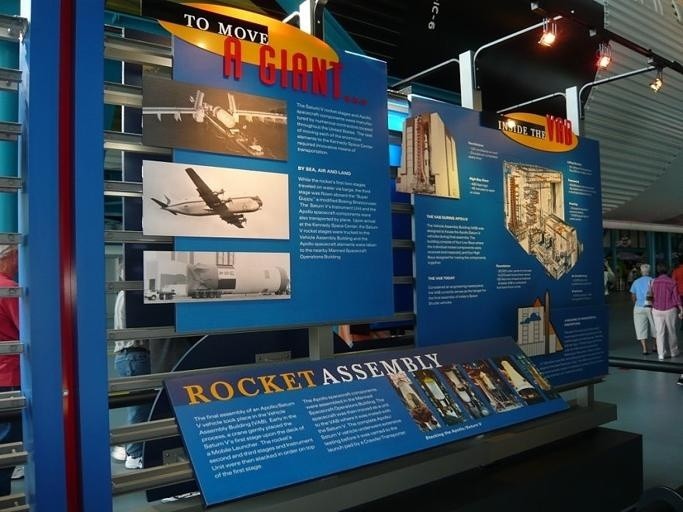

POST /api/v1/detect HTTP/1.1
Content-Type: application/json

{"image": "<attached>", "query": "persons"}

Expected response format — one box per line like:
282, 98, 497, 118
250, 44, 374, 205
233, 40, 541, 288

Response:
647, 261, 683, 360
671, 256, 683, 332
604, 259, 657, 355
0, 242, 23, 497
111, 265, 151, 469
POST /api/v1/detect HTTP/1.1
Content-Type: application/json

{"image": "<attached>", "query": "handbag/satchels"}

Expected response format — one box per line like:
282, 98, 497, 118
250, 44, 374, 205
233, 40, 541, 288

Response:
643, 294, 654, 309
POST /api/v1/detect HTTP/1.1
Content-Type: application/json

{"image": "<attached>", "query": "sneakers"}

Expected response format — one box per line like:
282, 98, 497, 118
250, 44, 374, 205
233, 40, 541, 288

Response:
641, 350, 683, 385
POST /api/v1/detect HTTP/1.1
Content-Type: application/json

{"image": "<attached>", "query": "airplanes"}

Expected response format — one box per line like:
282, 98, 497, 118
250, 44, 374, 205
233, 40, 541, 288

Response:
149, 168, 262, 228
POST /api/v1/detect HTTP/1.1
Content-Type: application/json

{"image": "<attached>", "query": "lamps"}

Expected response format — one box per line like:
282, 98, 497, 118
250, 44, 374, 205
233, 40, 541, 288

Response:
536, 13, 665, 94
